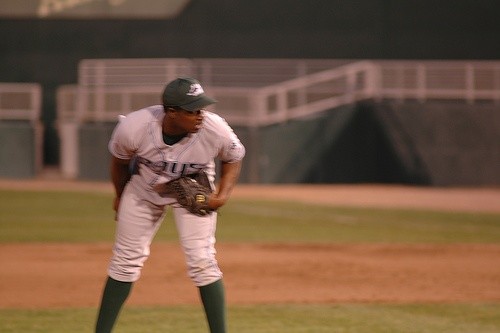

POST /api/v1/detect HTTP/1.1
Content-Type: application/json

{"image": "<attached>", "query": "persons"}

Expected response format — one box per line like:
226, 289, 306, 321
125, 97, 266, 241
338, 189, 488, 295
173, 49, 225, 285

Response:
95, 77, 246, 333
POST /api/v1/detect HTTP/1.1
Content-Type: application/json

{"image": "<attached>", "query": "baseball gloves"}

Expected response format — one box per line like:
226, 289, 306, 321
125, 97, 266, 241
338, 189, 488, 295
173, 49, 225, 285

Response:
164, 169, 211, 214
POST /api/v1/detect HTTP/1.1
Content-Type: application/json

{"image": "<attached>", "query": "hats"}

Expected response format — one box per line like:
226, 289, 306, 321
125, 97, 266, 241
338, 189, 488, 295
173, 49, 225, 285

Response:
162, 78, 219, 111
